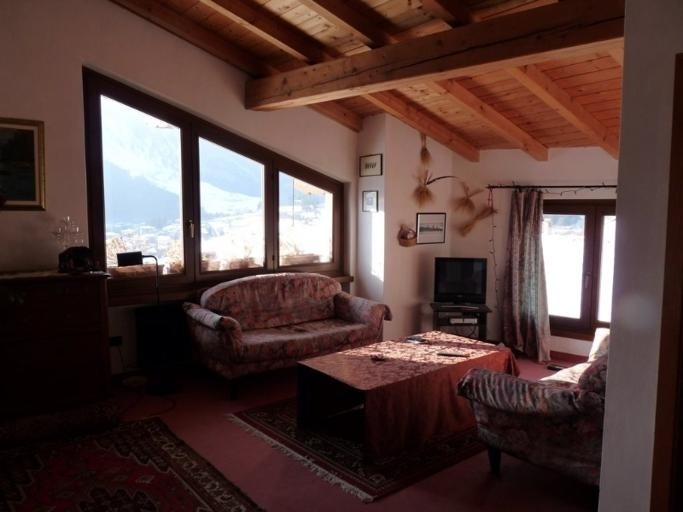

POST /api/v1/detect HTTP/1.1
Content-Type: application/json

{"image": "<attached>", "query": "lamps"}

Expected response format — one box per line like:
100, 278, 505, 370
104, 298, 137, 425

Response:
118, 253, 171, 397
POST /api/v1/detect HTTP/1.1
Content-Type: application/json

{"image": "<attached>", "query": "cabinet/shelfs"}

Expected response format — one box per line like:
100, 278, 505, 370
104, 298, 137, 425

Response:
430, 302, 492, 339
0, 267, 111, 404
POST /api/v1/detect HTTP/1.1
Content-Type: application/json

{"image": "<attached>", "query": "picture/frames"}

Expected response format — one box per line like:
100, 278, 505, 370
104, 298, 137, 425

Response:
415, 212, 446, 244
0, 116, 46, 212
359, 153, 382, 177
362, 191, 378, 212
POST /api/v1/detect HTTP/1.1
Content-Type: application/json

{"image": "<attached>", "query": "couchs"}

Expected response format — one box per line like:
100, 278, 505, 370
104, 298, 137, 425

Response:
183, 272, 393, 401
457, 328, 609, 485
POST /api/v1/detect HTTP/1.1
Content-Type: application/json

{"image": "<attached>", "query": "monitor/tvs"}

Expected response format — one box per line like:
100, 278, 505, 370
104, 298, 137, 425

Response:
434, 257, 487, 308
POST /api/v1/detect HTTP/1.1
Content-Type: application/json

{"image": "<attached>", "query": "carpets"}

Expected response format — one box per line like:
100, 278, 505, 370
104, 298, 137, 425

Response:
227, 405, 485, 503
1, 416, 267, 511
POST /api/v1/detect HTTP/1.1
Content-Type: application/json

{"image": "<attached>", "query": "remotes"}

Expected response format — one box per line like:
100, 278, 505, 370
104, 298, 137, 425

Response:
437, 351, 469, 356
